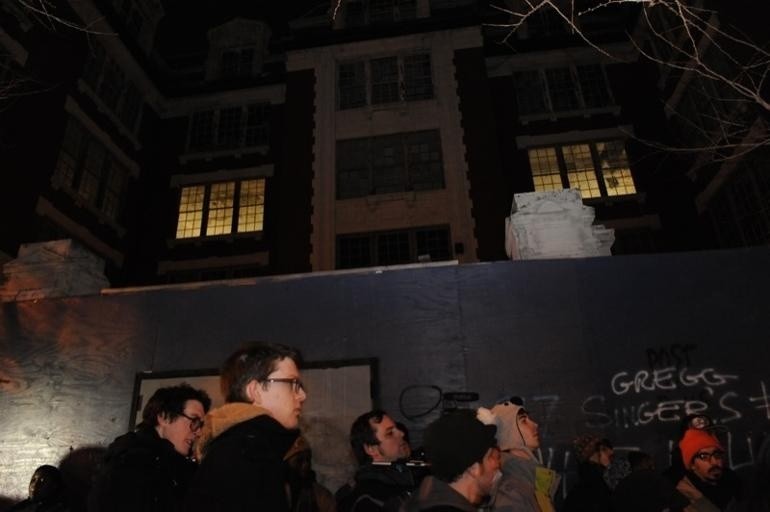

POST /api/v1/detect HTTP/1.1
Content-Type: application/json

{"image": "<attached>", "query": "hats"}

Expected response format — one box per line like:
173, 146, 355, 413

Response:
679, 427, 726, 472
423, 396, 529, 479
571, 432, 608, 463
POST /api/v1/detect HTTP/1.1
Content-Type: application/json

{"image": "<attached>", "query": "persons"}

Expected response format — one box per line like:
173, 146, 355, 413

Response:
336, 396, 554, 512
15, 385, 210, 512
194, 342, 318, 509
556, 427, 746, 512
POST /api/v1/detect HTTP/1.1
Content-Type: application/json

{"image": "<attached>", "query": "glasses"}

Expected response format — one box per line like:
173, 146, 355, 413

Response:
267, 378, 304, 394
696, 451, 723, 461
180, 413, 204, 432
